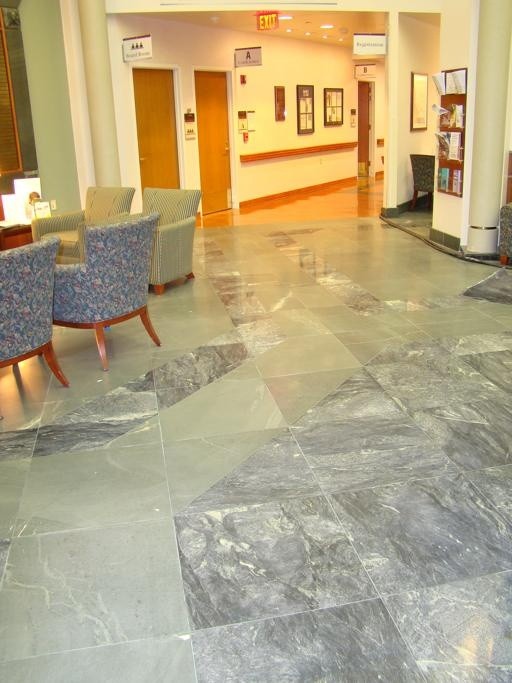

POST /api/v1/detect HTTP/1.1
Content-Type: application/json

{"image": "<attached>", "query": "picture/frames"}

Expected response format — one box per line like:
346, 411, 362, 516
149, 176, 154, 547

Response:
296, 86, 344, 134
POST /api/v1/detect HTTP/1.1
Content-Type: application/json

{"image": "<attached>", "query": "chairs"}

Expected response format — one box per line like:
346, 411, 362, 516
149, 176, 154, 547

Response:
408, 154, 438, 211
53, 212, 161, 372
2, 236, 70, 386
92, 186, 202, 294
32, 184, 135, 266
